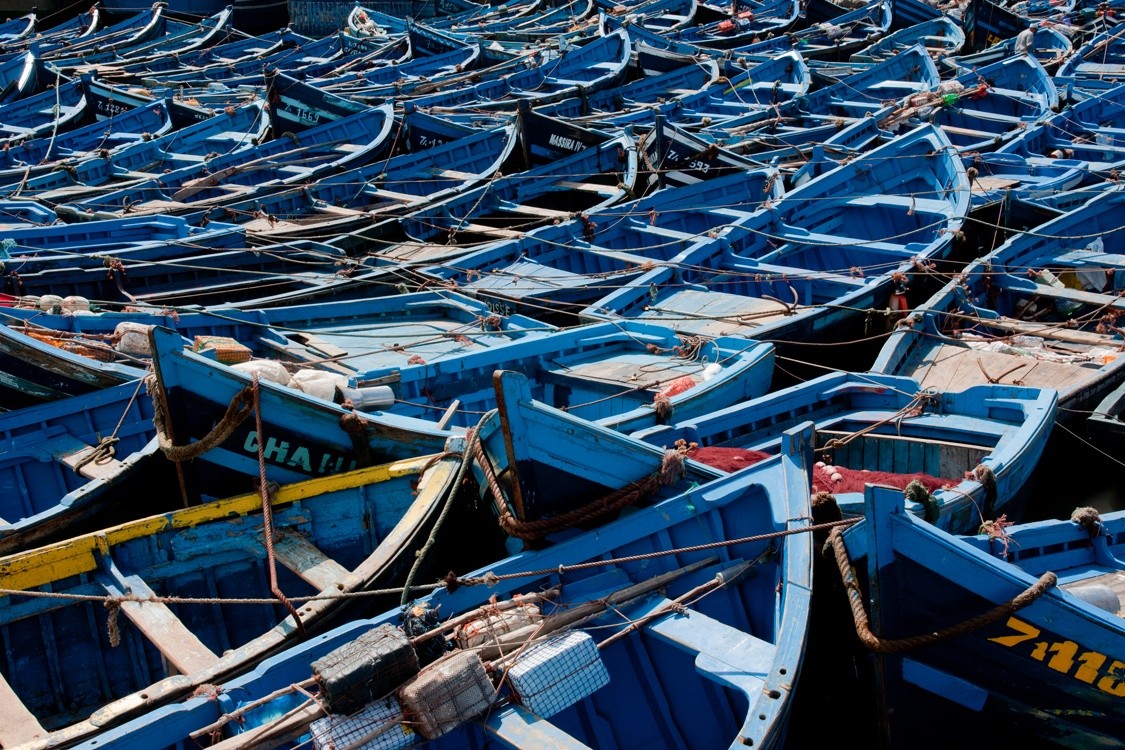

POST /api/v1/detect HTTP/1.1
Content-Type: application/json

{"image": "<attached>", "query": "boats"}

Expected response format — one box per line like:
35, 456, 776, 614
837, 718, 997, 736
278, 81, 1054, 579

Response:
0, 1, 1125, 750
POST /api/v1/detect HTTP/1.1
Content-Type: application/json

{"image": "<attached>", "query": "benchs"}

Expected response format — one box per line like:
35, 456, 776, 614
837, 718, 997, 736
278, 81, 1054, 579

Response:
256, 526, 352, 592
592, 582, 778, 681
721, 257, 869, 287
991, 277, 1125, 309
94, 568, 219, 674
38, 434, 122, 480
469, 692, 597, 750
960, 312, 1125, 348
0, 10, 1125, 271
